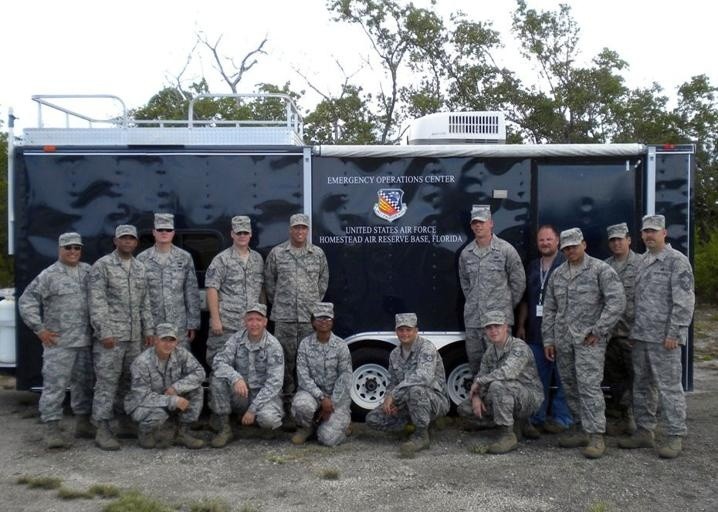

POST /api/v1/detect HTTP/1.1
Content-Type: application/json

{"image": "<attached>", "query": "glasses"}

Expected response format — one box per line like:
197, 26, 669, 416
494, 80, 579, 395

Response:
66, 246, 81, 250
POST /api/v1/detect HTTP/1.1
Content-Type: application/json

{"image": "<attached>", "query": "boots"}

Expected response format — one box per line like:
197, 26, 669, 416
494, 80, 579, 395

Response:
45, 421, 62, 448
402, 428, 430, 451
461, 420, 681, 457
75, 415, 312, 451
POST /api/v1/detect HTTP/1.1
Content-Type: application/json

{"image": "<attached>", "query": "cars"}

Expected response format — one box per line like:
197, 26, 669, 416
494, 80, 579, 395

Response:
0, 289, 16, 369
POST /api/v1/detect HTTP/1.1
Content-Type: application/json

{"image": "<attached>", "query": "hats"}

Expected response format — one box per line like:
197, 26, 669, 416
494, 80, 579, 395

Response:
395, 313, 417, 328
289, 214, 308, 227
606, 215, 665, 238
59, 233, 83, 246
116, 213, 174, 238
157, 323, 176, 339
312, 302, 334, 318
247, 303, 267, 317
470, 209, 491, 224
480, 311, 505, 327
232, 216, 251, 233
559, 227, 584, 250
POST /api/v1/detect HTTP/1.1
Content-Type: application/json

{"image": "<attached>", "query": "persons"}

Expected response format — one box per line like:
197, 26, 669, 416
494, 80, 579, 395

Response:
366, 312, 450, 452
617, 214, 695, 459
263, 213, 329, 420
541, 228, 627, 458
469, 311, 546, 454
458, 204, 540, 439
136, 213, 204, 430
88, 224, 155, 450
124, 322, 207, 448
516, 223, 575, 433
289, 302, 352, 448
601, 222, 638, 436
209, 304, 284, 448
18, 232, 96, 448
204, 216, 267, 368
